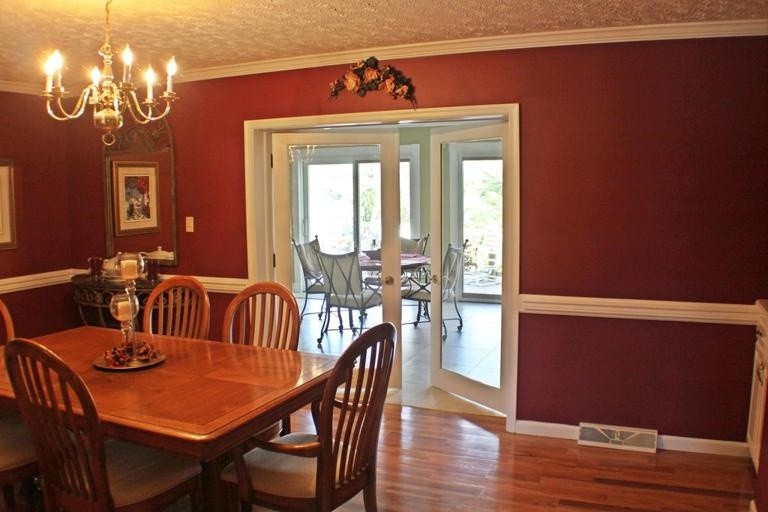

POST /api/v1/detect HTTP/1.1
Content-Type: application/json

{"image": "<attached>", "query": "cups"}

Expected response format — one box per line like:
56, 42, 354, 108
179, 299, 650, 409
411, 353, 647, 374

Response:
87, 251, 145, 284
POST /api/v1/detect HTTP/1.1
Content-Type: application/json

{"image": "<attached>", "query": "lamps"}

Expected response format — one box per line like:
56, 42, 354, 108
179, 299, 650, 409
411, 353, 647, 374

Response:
40, 1, 179, 145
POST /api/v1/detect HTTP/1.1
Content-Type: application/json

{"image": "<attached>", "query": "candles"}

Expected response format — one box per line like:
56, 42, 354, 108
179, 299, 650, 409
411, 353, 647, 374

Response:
118, 301, 131, 320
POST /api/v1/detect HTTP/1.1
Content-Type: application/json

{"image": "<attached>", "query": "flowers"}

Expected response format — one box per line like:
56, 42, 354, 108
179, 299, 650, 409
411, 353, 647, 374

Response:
325, 56, 420, 116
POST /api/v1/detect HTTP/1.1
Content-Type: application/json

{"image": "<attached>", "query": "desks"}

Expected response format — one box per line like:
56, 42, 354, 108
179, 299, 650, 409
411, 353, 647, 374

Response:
73, 274, 195, 333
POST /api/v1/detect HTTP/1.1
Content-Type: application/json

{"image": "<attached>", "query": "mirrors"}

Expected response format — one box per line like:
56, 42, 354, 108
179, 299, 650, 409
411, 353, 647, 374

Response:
101, 106, 178, 268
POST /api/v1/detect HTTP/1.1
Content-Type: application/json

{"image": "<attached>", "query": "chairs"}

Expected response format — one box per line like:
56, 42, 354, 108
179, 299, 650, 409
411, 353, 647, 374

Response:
220, 281, 301, 436
2, 338, 204, 512
293, 231, 468, 343
0, 412, 82, 512
0, 298, 13, 342
214, 321, 396, 511
142, 276, 210, 342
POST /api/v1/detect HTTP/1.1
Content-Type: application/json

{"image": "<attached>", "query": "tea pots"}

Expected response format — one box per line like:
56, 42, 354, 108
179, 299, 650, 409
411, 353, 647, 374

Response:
140, 245, 174, 261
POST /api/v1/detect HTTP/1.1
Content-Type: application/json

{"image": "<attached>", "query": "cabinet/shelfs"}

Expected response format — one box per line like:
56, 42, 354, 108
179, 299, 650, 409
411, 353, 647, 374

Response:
744, 321, 768, 472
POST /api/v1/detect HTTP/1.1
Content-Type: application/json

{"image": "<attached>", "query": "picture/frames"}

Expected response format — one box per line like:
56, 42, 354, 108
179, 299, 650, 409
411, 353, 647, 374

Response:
1, 158, 17, 251
112, 162, 160, 237
